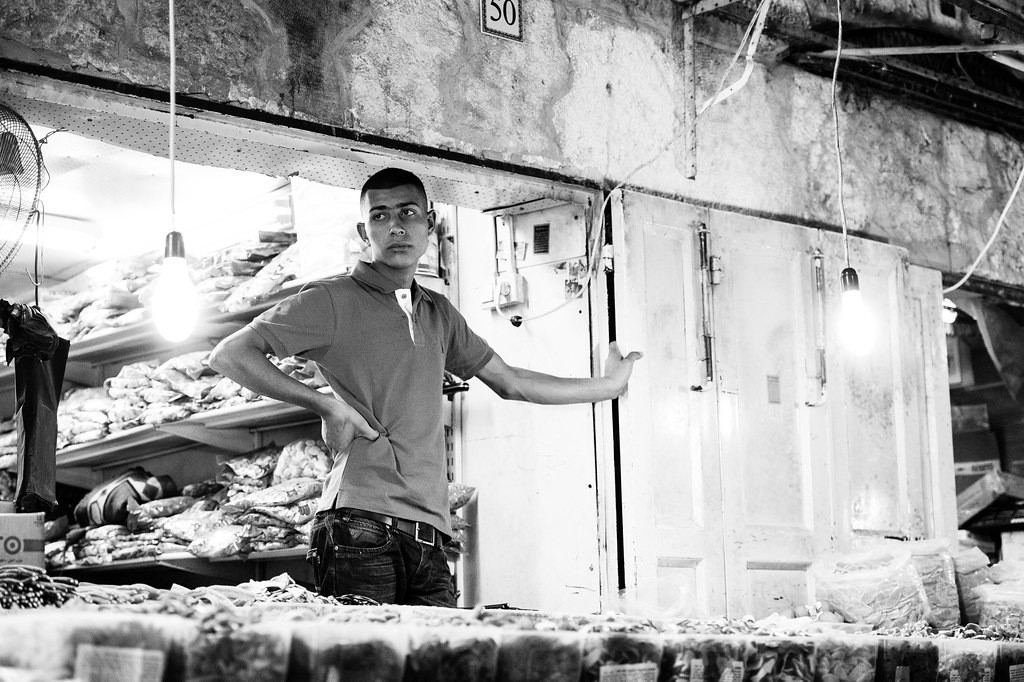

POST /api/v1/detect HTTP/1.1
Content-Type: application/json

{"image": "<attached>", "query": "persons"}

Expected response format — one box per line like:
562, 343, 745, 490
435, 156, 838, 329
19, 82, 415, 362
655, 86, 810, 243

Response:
208, 168, 644, 609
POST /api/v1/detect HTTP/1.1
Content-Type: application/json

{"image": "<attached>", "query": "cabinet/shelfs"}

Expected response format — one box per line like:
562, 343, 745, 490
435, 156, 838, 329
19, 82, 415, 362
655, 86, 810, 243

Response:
0, 271, 465, 578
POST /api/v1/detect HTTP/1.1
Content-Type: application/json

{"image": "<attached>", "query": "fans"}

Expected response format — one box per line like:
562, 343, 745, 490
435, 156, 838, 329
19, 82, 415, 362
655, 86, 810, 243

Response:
0, 105, 43, 277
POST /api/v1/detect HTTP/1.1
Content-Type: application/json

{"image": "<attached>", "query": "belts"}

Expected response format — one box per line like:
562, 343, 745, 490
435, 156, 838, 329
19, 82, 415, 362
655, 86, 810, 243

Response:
336, 507, 442, 548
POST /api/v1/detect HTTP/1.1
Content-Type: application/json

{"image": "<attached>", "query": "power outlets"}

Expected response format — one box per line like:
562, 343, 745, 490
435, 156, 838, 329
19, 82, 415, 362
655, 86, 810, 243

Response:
484, 273, 525, 311
